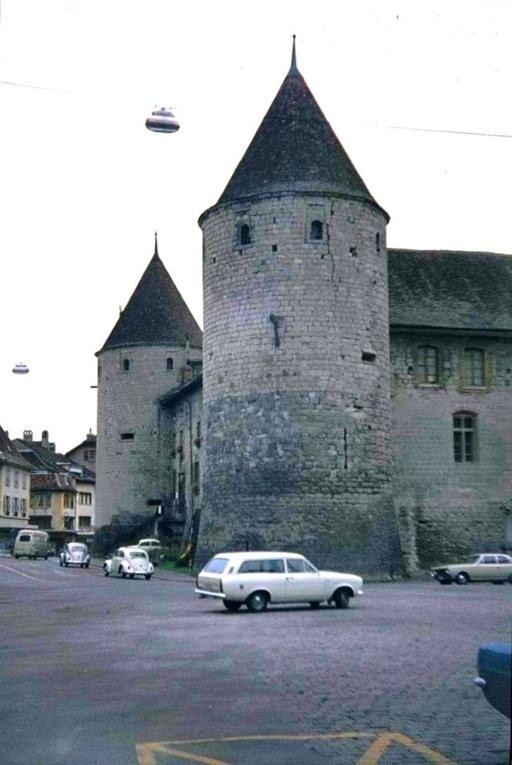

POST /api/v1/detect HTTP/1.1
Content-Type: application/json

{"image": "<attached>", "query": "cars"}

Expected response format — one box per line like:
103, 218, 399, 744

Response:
102, 538, 170, 580
194, 550, 364, 612
429, 553, 512, 585
59, 542, 91, 568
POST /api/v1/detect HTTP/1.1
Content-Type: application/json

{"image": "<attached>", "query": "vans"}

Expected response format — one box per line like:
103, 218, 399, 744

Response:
12, 529, 50, 561
